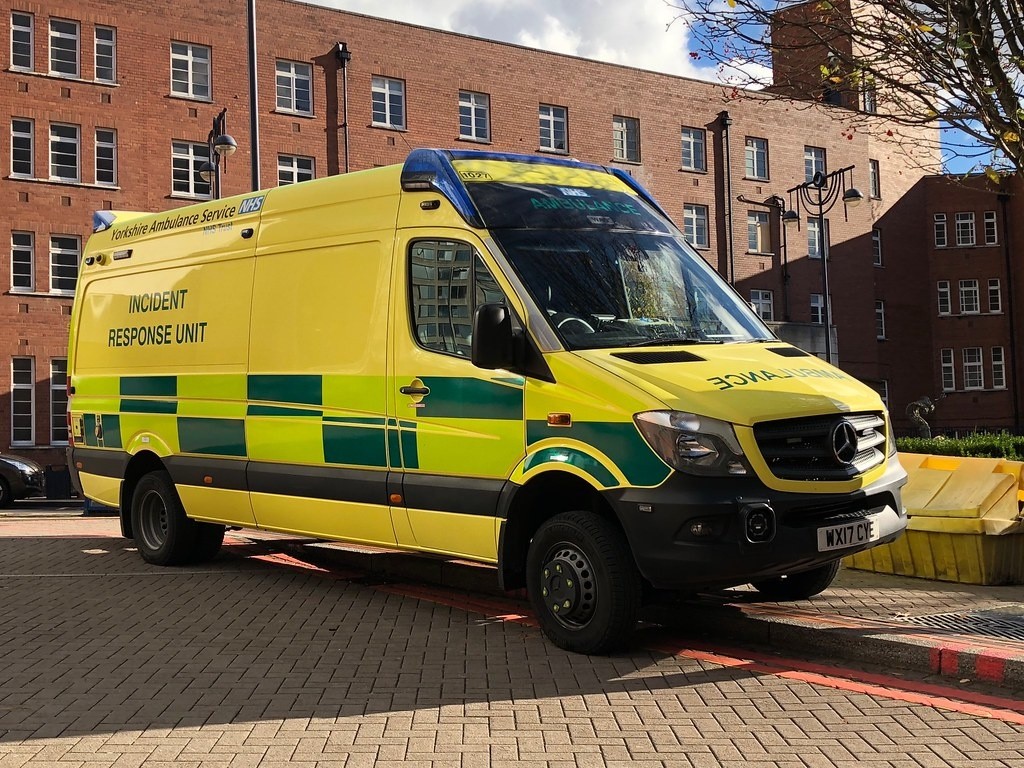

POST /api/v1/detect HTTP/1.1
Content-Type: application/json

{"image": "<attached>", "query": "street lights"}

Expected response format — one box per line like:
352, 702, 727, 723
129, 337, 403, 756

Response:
199, 107, 237, 200
781, 165, 863, 366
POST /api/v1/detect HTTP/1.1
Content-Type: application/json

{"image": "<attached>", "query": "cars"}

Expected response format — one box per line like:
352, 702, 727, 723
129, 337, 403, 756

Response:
0, 454, 44, 510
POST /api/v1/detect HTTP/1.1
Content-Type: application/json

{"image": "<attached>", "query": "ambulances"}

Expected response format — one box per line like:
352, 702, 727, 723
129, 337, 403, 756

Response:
64, 150, 908, 656
